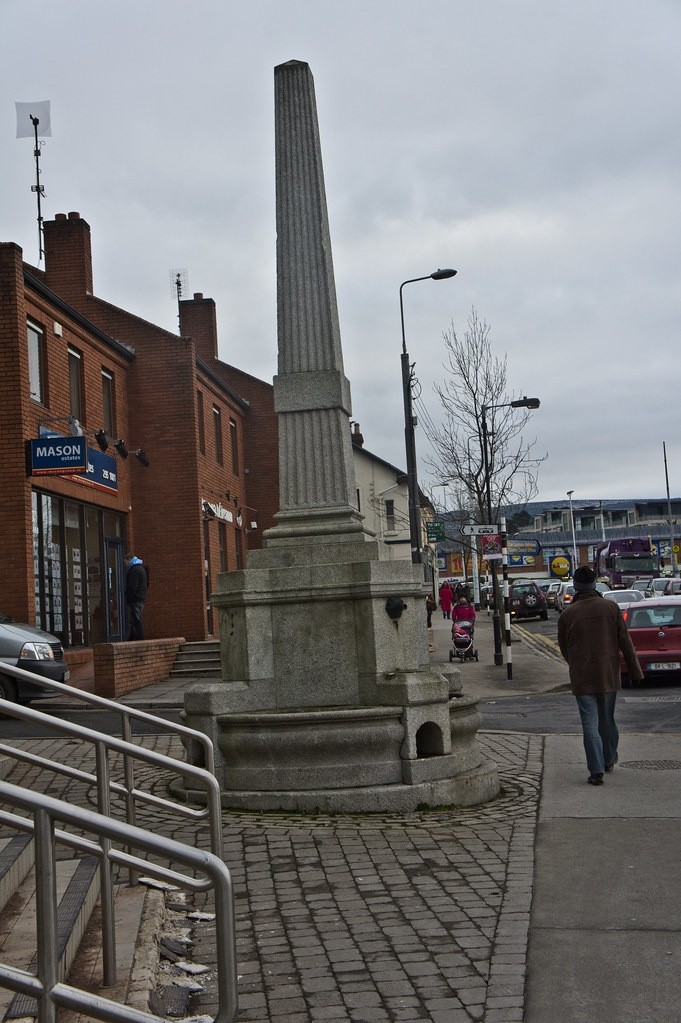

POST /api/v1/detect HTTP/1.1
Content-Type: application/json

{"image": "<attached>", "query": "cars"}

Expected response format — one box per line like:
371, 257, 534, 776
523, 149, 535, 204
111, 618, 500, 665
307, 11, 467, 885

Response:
0, 612, 70, 720
536, 579, 573, 614
448, 579, 511, 612
601, 589, 646, 615
568, 584, 616, 605
619, 599, 681, 689
632, 595, 681, 624
630, 576, 681, 598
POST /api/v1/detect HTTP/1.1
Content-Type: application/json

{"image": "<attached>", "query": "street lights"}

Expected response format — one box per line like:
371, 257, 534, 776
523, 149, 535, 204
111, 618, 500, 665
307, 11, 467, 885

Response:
397, 265, 460, 564
430, 483, 448, 504
478, 397, 542, 666
465, 431, 499, 611
565, 489, 579, 568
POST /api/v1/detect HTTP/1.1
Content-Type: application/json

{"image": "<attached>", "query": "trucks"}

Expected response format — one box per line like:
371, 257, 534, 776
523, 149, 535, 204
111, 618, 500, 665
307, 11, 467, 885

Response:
594, 534, 666, 591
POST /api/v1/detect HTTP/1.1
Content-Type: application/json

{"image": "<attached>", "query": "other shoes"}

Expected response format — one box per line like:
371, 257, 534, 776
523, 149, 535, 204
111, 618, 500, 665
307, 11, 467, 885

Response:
605, 752, 619, 771
586, 771, 603, 787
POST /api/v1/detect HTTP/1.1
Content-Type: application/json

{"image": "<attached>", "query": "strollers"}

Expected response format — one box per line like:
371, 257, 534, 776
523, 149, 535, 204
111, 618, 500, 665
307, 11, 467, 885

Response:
448, 619, 480, 662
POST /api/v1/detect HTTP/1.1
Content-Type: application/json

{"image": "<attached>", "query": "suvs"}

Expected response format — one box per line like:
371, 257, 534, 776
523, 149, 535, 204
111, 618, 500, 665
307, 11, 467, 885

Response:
508, 583, 549, 622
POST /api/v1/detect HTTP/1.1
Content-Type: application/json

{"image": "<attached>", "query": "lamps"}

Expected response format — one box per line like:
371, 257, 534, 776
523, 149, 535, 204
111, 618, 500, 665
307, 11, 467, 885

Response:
39, 416, 150, 468
202, 485, 260, 533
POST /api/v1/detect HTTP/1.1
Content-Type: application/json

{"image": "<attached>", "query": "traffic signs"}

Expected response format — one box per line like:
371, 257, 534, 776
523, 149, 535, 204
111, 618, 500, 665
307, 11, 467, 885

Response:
459, 523, 500, 536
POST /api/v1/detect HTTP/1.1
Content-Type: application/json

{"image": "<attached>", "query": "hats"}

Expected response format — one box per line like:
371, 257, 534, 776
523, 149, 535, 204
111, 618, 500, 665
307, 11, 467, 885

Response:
572, 565, 596, 591
452, 623, 459, 630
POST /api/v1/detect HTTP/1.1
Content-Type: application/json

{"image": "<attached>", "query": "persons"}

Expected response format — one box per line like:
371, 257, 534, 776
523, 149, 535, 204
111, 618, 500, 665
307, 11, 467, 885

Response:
124, 552, 148, 640
440, 581, 471, 620
556, 566, 644, 784
451, 595, 476, 626
452, 622, 469, 638
